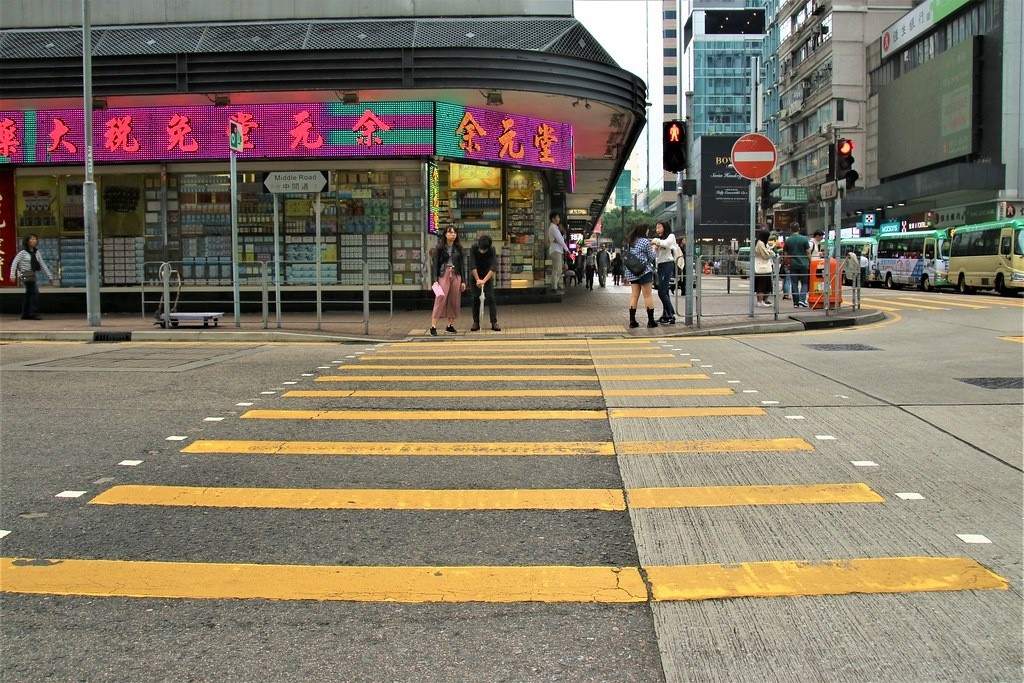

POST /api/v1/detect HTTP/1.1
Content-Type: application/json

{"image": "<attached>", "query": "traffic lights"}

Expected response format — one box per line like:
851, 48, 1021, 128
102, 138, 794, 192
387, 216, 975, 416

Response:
761, 180, 783, 209
662, 120, 688, 174
836, 137, 859, 190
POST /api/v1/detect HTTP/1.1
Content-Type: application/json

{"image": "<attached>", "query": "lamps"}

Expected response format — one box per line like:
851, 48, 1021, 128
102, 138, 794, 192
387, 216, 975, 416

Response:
486, 90, 503, 106
93, 96, 107, 111
214, 93, 231, 106
887, 202, 895, 208
898, 200, 906, 206
846, 209, 854, 218
338, 91, 360, 105
572, 99, 582, 107
876, 207, 884, 210
603, 113, 623, 160
857, 210, 864, 213
854, 209, 861, 216
585, 98, 592, 109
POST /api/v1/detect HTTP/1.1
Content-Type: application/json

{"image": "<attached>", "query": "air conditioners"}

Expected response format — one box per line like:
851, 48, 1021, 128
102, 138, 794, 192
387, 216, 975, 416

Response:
786, 144, 792, 155
797, 82, 809, 89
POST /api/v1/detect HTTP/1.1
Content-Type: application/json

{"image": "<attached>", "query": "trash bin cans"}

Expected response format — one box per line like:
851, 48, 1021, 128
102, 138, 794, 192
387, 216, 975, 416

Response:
705, 266, 710, 274
808, 259, 844, 309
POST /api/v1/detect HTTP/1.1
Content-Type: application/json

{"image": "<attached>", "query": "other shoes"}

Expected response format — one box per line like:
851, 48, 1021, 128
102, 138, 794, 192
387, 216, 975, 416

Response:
654, 316, 664, 323
471, 323, 480, 331
794, 305, 798, 308
757, 300, 770, 307
551, 288, 567, 295
590, 288, 593, 290
492, 323, 501, 331
430, 327, 438, 336
783, 296, 791, 300
798, 301, 808, 307
765, 301, 773, 306
681, 291, 685, 296
586, 285, 589, 289
660, 316, 676, 324
445, 325, 457, 333
21, 315, 42, 320
670, 284, 675, 294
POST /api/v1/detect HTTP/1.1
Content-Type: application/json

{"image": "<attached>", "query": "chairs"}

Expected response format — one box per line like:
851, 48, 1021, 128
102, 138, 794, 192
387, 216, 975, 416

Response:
974, 238, 998, 256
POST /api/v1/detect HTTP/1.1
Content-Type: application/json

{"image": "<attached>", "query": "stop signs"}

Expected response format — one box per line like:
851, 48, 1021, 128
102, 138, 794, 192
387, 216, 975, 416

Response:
731, 132, 777, 181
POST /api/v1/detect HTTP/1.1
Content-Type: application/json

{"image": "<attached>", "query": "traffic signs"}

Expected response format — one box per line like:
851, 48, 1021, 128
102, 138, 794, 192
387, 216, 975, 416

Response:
264, 171, 328, 193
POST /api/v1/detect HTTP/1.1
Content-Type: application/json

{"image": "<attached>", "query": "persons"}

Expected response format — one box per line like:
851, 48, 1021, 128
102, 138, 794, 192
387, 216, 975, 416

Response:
567, 246, 623, 290
755, 230, 776, 307
860, 254, 869, 287
669, 237, 686, 296
625, 220, 677, 328
714, 260, 719, 275
470, 235, 501, 331
782, 222, 825, 307
430, 225, 466, 336
549, 213, 571, 293
11, 233, 53, 320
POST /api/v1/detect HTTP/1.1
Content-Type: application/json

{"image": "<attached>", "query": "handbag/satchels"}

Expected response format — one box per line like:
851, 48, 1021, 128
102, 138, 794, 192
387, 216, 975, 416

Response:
612, 258, 617, 267
624, 253, 647, 277
755, 256, 772, 273
21, 271, 35, 281
779, 265, 787, 279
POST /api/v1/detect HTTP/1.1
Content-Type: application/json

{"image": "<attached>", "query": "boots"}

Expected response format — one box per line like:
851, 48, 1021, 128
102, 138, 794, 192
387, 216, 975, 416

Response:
629, 306, 639, 328
646, 307, 658, 328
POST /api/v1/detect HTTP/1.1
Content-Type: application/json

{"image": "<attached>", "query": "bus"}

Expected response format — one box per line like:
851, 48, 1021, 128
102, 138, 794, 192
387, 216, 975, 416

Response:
735, 235, 880, 287
873, 227, 956, 293
947, 218, 1024, 297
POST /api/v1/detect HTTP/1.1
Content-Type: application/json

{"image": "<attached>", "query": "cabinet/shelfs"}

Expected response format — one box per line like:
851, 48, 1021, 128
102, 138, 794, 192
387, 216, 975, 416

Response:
13, 169, 424, 288
453, 187, 503, 255
506, 200, 546, 241
